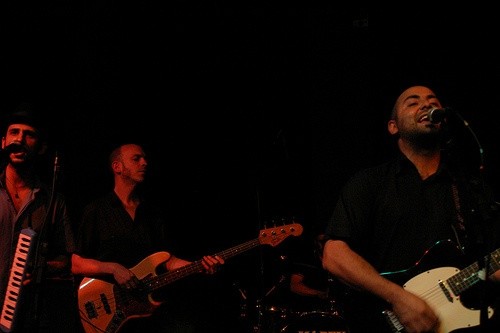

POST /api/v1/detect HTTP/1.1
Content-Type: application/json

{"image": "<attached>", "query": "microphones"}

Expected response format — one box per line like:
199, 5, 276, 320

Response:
54, 149, 62, 173
428, 107, 453, 124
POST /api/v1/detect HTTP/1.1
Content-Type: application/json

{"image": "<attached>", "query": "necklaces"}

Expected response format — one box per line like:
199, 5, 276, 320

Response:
5, 176, 28, 198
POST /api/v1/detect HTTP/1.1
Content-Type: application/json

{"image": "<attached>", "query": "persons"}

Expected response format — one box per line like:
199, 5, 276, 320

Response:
0, 104, 74, 332
70, 138, 226, 333
290, 231, 331, 298
320, 81, 500, 332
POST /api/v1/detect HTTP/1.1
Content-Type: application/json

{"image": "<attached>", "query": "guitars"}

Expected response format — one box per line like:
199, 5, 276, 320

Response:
333, 239, 500, 333
77, 214, 303, 333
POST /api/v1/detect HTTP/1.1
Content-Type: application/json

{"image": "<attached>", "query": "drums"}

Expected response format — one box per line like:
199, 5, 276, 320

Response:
295, 310, 349, 333
238, 303, 290, 333
